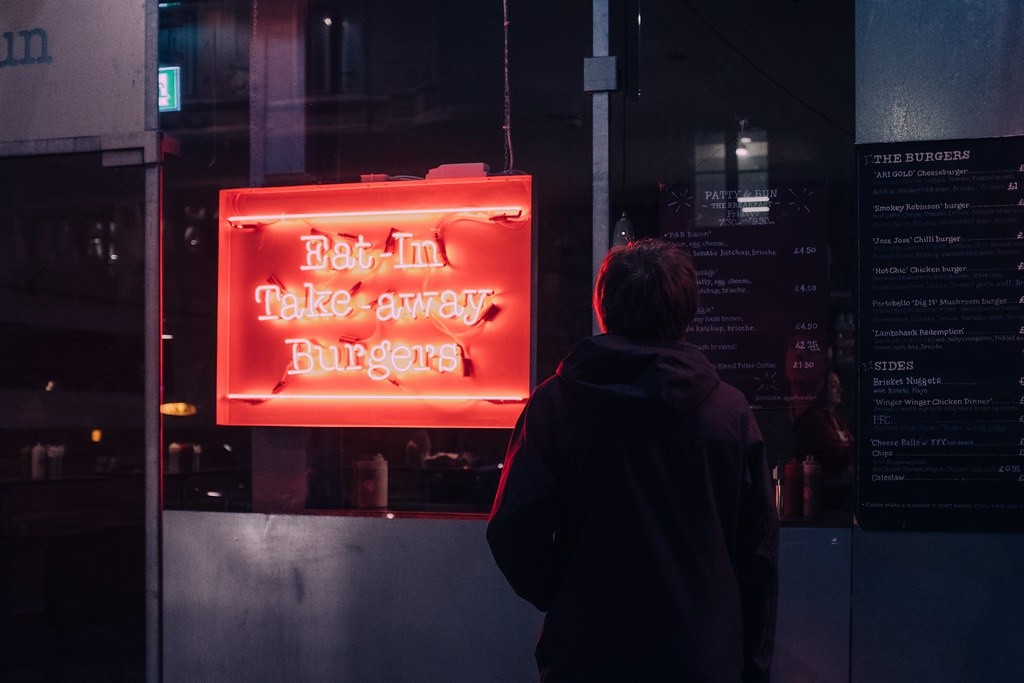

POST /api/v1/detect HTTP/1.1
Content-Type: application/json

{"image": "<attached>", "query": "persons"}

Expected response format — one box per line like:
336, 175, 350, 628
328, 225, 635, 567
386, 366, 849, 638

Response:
486, 239, 781, 683
757, 321, 856, 521
406, 429, 475, 468
